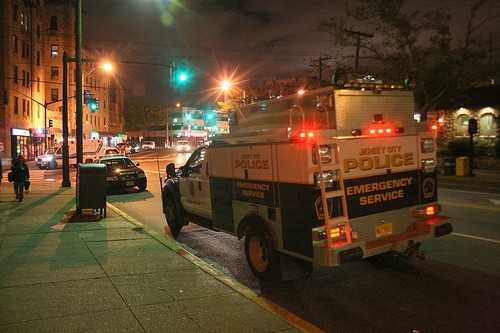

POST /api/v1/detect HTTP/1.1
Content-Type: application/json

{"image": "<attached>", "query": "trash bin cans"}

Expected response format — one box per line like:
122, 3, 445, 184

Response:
443, 158, 455, 176
76, 162, 108, 218
456, 157, 469, 176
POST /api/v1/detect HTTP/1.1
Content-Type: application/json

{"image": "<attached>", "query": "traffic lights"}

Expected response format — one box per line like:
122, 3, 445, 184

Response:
208, 114, 212, 119
187, 113, 190, 123
3, 91, 8, 104
49, 119, 53, 128
181, 74, 187, 81
90, 101, 96, 113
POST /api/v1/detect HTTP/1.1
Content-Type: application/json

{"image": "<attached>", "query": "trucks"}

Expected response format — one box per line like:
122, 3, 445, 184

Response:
35, 139, 104, 169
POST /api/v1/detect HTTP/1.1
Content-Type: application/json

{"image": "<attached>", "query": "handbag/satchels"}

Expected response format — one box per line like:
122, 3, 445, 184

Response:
8, 172, 14, 182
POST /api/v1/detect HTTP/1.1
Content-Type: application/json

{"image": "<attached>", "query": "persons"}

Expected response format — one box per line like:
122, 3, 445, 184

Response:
11, 155, 30, 202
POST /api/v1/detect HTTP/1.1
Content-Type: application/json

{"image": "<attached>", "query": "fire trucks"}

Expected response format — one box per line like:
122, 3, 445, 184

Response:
159, 82, 453, 280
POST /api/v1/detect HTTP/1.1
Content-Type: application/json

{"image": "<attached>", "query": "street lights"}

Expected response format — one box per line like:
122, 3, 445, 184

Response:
166, 103, 180, 148
223, 81, 245, 106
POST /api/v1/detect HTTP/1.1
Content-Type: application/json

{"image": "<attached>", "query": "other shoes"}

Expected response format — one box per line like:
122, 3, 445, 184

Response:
19, 200, 22, 202
16, 196, 18, 200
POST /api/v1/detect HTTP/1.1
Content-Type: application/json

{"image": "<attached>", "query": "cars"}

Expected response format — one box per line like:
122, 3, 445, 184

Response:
117, 142, 140, 154
98, 156, 147, 191
142, 140, 155, 151
104, 148, 119, 155
176, 140, 190, 152
